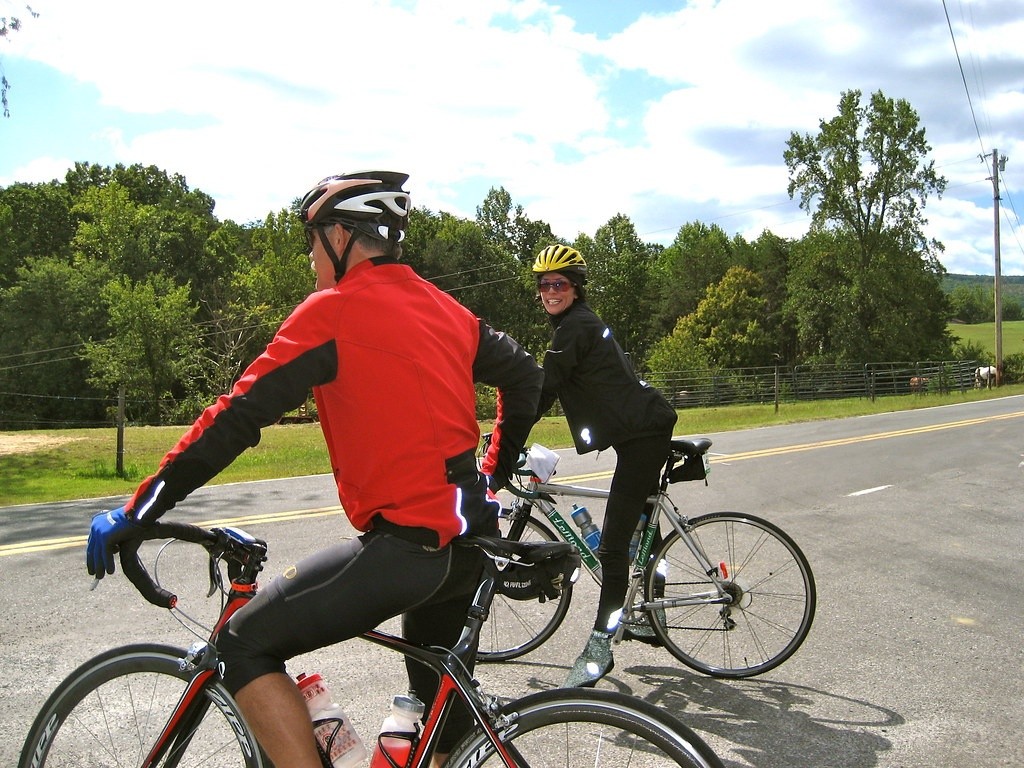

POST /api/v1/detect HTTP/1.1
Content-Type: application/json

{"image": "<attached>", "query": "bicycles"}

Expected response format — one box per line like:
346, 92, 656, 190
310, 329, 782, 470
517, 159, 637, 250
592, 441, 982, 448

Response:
13, 508, 734, 768
419, 427, 818, 681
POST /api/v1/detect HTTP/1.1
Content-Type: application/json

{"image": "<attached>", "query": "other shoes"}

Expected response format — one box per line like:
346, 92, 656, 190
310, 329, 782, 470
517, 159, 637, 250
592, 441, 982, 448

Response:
559, 633, 615, 693
622, 602, 668, 648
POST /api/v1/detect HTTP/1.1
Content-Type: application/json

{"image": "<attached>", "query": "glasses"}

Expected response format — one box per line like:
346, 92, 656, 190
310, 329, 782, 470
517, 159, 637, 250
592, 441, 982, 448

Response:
304, 227, 316, 249
538, 280, 577, 292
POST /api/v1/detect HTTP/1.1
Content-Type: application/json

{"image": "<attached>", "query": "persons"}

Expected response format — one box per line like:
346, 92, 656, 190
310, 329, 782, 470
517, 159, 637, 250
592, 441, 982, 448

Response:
532, 244, 677, 689
87, 172, 546, 767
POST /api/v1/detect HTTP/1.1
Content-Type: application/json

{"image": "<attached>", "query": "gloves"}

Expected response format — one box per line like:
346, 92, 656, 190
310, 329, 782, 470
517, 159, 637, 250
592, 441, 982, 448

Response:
85, 507, 133, 578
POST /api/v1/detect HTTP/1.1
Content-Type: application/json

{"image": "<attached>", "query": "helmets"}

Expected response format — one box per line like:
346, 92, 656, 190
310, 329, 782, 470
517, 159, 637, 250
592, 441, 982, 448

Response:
534, 244, 588, 284
298, 171, 410, 243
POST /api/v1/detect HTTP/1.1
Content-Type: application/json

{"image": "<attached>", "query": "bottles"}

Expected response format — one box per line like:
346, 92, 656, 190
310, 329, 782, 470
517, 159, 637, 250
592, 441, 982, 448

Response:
570, 503, 603, 559
368, 690, 425, 768
294, 672, 370, 768
626, 512, 647, 566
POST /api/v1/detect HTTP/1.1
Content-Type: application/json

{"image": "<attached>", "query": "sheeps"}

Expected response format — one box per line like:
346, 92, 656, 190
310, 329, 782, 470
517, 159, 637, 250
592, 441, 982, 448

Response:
910, 377, 930, 395
974, 366, 1002, 389
